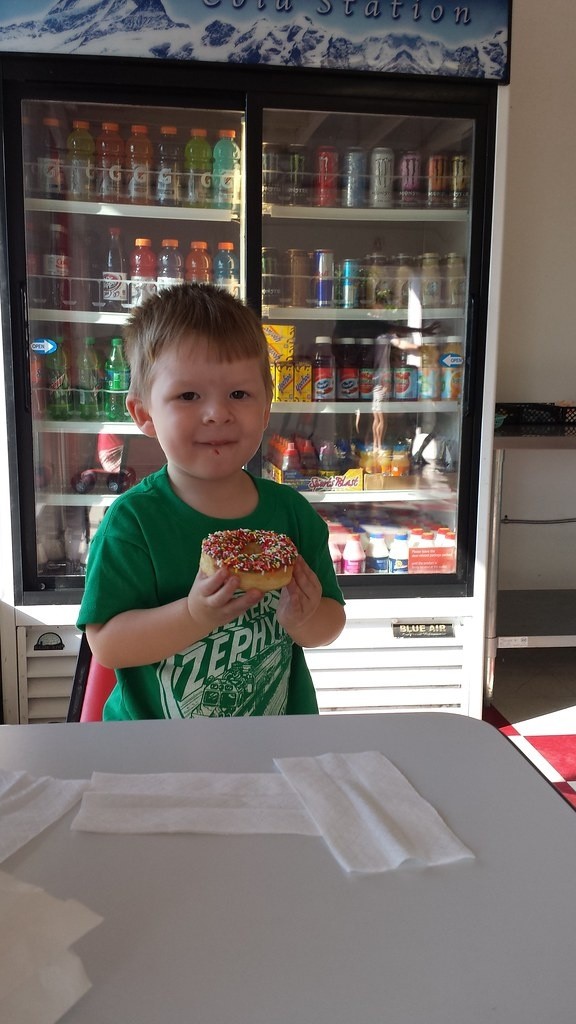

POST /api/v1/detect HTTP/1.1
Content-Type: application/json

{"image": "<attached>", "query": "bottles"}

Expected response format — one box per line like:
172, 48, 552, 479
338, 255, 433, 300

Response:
27, 222, 43, 310
65, 121, 95, 202
22, 117, 38, 199
317, 506, 456, 575
212, 243, 240, 302
102, 229, 130, 312
313, 334, 465, 403
185, 240, 212, 287
43, 223, 68, 310
29, 336, 133, 421
213, 129, 242, 211
95, 121, 126, 203
155, 126, 182, 206
184, 128, 213, 208
268, 432, 410, 478
37, 118, 66, 199
129, 238, 156, 309
126, 124, 156, 205
157, 239, 184, 297
367, 252, 466, 309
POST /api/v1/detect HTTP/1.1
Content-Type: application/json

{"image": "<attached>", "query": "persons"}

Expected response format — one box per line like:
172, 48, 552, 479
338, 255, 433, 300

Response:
77, 285, 346, 720
330, 239, 439, 451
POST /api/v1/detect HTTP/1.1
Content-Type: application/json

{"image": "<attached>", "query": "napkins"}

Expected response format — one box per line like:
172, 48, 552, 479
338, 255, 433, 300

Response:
0, 869, 103, 1024
0, 769, 92, 865
271, 749, 476, 876
70, 771, 322, 837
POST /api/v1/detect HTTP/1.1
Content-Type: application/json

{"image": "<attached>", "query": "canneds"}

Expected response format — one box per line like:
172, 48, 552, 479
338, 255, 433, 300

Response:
260, 141, 472, 212
260, 246, 361, 310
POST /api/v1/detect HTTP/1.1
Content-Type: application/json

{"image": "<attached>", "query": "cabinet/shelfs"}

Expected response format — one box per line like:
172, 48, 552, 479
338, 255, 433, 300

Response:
484, 421, 576, 709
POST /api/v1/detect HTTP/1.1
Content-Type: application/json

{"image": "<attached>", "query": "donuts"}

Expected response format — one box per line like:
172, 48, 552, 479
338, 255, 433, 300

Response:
200, 527, 298, 592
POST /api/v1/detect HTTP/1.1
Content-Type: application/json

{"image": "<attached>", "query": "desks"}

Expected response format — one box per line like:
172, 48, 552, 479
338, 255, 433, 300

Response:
0, 713, 576, 1024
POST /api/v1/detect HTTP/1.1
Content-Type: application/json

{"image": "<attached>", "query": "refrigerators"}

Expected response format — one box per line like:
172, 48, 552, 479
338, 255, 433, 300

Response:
0, 0, 513, 719
